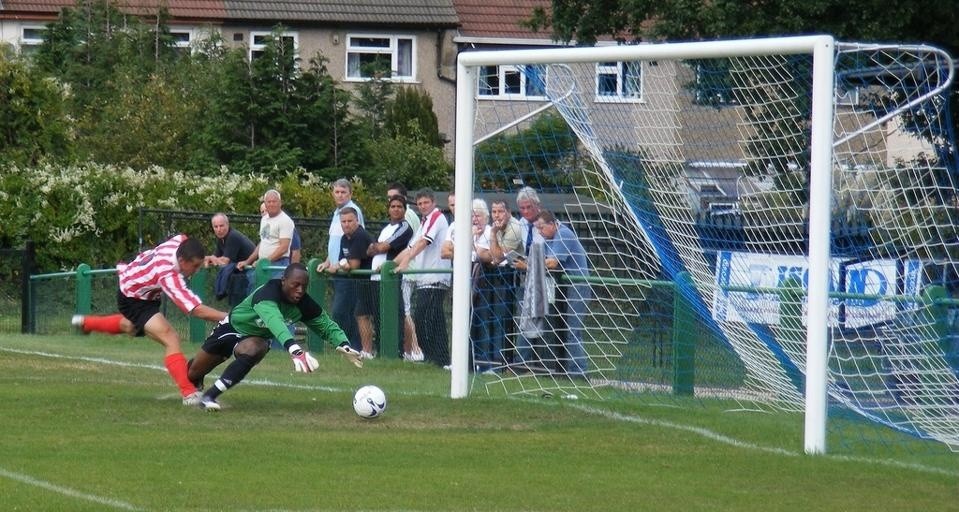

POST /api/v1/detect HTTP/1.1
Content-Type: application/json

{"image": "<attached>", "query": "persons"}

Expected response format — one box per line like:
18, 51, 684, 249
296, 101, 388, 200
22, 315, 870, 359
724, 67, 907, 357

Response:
182, 262, 366, 411
201, 211, 256, 308
253, 190, 298, 348
316, 176, 592, 379
70, 232, 232, 408
236, 202, 302, 350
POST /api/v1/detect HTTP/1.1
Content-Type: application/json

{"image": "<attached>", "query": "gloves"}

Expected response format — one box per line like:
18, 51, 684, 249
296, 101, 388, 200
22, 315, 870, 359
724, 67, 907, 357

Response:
288, 343, 319, 374
335, 342, 364, 369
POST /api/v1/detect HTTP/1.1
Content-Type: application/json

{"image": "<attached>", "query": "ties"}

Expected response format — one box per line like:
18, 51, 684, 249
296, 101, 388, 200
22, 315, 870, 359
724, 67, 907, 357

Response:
526, 223, 534, 256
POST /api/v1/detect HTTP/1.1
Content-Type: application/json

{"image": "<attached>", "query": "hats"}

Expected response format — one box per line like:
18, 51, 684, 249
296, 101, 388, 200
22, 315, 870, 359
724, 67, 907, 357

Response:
388, 195, 406, 210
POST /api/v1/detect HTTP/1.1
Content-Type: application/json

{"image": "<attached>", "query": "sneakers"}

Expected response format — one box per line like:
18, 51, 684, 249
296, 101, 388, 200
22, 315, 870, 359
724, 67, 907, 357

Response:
71, 314, 90, 335
182, 391, 205, 407
200, 395, 222, 412
359, 347, 505, 376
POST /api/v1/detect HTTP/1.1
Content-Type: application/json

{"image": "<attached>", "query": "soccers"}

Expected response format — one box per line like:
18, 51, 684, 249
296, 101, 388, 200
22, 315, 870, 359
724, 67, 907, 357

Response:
352, 385, 386, 419
